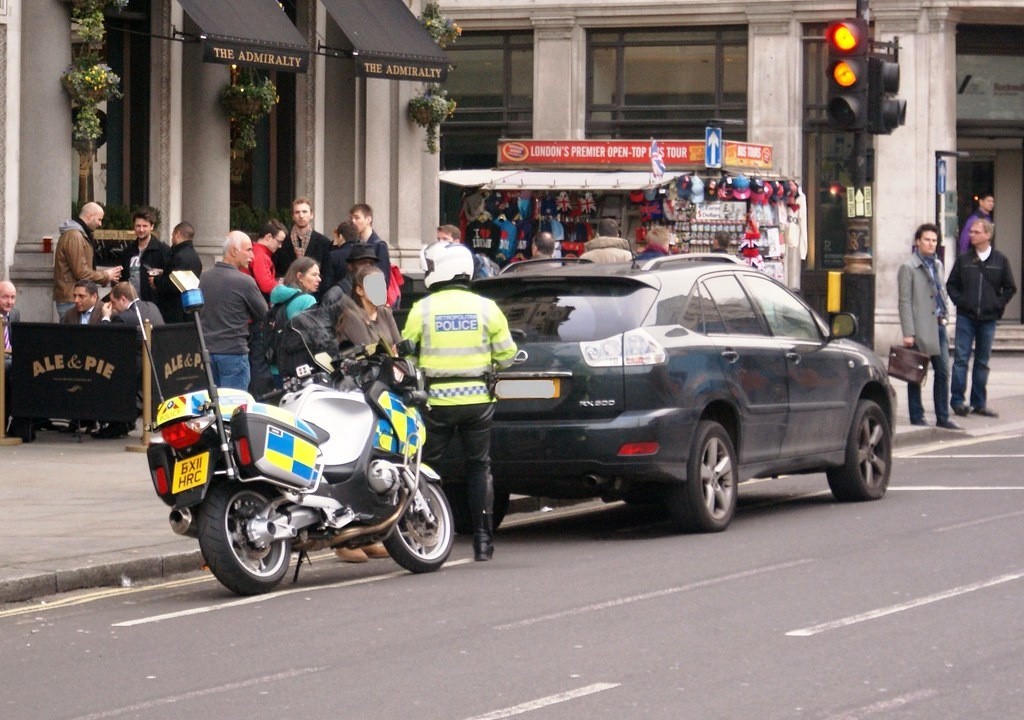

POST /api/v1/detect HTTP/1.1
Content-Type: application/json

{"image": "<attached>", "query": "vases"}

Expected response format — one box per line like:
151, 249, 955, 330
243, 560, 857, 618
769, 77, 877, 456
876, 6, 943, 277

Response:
73, 0, 106, 9
411, 108, 433, 124
437, 33, 451, 42
65, 80, 104, 102
230, 99, 261, 115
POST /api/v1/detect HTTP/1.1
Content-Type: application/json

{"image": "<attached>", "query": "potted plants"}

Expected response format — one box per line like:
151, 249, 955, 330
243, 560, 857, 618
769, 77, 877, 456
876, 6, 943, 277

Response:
229, 201, 292, 242
71, 199, 161, 240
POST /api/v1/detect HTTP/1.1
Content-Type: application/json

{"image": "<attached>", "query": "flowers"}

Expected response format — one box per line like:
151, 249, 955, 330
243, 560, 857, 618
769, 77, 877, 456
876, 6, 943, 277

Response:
219, 76, 279, 153
417, 3, 462, 48
61, 0, 129, 141
408, 81, 457, 156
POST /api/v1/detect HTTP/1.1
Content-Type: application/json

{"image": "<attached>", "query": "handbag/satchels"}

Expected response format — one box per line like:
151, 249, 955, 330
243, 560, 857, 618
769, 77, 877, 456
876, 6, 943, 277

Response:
887, 342, 930, 384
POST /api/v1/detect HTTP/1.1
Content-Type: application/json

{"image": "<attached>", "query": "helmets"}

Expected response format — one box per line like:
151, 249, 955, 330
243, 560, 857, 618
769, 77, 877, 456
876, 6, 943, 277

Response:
424, 240, 474, 291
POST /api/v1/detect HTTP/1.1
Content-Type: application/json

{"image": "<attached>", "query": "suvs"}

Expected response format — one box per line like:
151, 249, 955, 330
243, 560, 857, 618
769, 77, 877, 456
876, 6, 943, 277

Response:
444, 251, 896, 533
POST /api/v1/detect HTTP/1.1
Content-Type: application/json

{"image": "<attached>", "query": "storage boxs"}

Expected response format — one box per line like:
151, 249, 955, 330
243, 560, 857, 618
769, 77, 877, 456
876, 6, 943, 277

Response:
145, 443, 178, 507
229, 404, 330, 487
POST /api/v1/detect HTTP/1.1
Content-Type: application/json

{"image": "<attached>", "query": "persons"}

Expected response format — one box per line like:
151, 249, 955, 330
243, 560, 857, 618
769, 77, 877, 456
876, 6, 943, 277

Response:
197, 197, 390, 390
59, 280, 104, 326
946, 219, 1017, 416
54, 202, 122, 320
898, 223, 962, 430
711, 231, 729, 253
101, 282, 165, 326
111, 208, 170, 302
437, 225, 461, 243
577, 217, 632, 264
514, 231, 561, 273
0, 280, 19, 366
396, 240, 517, 560
634, 226, 672, 261
337, 266, 401, 563
959, 192, 995, 256
141, 221, 203, 323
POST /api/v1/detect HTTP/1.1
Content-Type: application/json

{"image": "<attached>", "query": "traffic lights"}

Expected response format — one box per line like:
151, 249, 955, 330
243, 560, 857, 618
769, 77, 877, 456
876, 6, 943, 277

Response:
827, 271, 845, 314
871, 55, 908, 135
824, 19, 869, 131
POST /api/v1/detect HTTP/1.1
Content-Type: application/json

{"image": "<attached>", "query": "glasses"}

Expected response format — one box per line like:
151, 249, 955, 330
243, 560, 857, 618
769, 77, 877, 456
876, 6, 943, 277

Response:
969, 230, 987, 237
274, 236, 282, 244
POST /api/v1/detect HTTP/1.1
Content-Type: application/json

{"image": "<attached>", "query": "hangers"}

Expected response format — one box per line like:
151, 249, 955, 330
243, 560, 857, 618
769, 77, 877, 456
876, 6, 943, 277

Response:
478, 212, 562, 223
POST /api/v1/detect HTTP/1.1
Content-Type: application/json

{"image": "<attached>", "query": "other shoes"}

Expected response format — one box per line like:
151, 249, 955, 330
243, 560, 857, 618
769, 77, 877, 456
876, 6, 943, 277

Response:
976, 406, 997, 417
914, 419, 930, 426
361, 541, 390, 558
935, 418, 959, 429
954, 404, 974, 416
89, 420, 134, 439
34, 418, 57, 431
335, 546, 369, 562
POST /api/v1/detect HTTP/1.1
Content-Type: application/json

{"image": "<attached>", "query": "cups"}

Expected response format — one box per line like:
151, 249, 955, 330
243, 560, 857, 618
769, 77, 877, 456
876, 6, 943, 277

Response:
43, 237, 52, 253
148, 270, 159, 287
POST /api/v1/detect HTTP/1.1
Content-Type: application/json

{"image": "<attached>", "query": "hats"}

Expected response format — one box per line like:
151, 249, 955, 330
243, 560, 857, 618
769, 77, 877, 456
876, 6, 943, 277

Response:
345, 243, 381, 263
631, 174, 799, 207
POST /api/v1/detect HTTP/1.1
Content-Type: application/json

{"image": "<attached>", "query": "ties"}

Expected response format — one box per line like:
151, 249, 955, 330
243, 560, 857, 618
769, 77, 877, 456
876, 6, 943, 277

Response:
4, 316, 11, 350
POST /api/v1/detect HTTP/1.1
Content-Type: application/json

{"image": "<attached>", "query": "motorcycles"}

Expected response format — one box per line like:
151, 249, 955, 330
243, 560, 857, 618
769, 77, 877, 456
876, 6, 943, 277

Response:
146, 270, 455, 595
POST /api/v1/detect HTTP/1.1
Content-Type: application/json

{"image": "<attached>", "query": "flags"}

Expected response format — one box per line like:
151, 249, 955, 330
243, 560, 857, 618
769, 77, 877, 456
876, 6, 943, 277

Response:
649, 141, 665, 179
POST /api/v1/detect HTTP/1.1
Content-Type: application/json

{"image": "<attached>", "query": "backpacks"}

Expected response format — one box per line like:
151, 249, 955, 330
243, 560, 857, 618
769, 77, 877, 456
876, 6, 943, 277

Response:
260, 291, 302, 365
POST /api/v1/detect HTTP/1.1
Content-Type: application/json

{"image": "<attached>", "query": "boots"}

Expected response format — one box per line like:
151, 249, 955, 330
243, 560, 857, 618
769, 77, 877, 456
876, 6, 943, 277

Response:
469, 474, 494, 561
